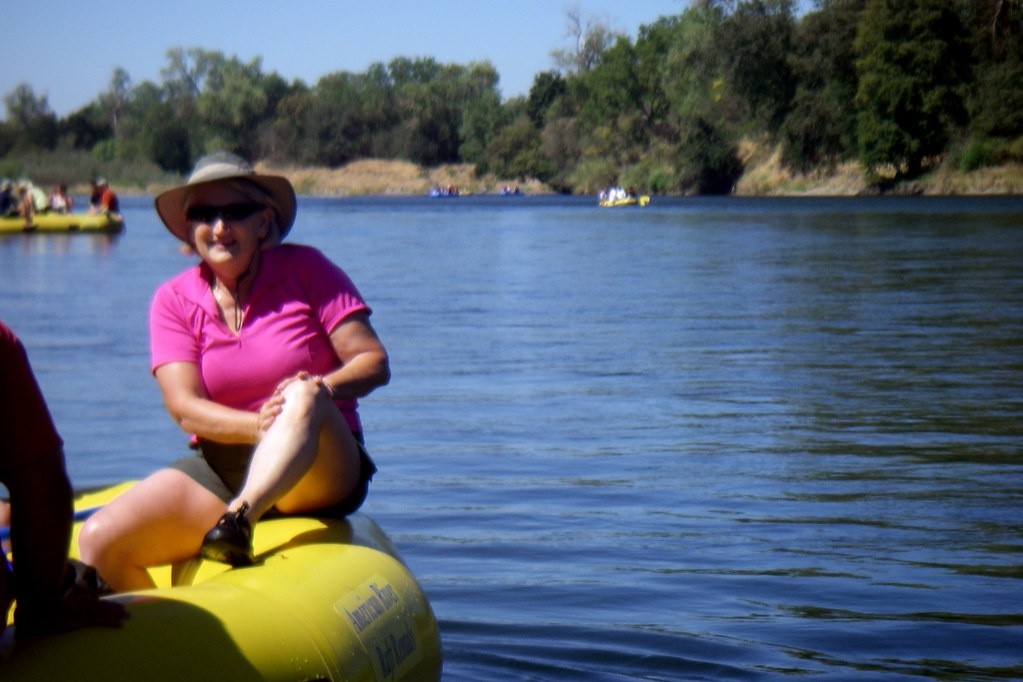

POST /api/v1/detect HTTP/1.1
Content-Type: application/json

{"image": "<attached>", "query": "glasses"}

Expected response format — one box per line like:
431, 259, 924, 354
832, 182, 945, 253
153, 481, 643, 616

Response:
185, 203, 263, 223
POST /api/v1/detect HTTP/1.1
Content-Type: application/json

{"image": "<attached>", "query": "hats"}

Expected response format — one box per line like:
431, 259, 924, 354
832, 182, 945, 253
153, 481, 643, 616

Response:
94, 178, 109, 187
152, 153, 297, 253
0, 179, 16, 193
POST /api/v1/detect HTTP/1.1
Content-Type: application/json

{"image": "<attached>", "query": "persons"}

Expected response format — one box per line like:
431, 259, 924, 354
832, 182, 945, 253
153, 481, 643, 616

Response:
599, 185, 636, 202
0, 319, 128, 645
0, 176, 73, 229
89, 175, 119, 216
500, 184, 522, 194
78, 153, 390, 594
429, 184, 469, 195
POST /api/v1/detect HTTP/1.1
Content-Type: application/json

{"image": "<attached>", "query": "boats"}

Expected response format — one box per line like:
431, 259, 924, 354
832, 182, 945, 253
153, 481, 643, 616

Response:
0, 478, 444, 682
0, 205, 124, 237
600, 194, 638, 209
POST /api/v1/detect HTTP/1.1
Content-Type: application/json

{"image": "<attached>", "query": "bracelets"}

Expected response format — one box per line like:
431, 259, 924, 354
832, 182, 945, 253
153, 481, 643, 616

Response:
317, 376, 334, 399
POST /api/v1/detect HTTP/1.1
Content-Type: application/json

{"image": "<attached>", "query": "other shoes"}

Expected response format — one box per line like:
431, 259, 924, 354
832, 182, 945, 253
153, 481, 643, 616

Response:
198, 511, 255, 564
